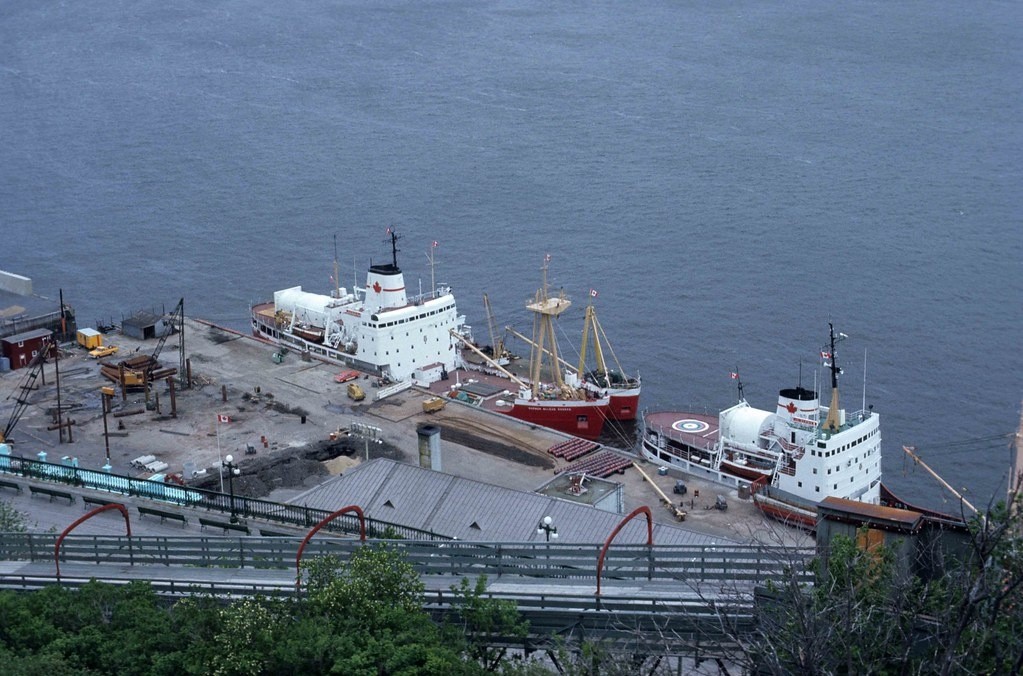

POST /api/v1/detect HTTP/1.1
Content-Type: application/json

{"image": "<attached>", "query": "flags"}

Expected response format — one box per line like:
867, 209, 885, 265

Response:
218, 415, 230, 423
820, 351, 831, 358
729, 372, 738, 379
590, 288, 597, 297
432, 240, 438, 247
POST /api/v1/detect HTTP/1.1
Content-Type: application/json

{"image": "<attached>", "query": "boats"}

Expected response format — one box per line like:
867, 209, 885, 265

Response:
640, 319, 973, 531
577, 288, 641, 421
247, 223, 611, 444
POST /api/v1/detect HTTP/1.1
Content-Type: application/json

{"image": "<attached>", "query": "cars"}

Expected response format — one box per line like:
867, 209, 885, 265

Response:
334, 371, 359, 383
88, 345, 119, 359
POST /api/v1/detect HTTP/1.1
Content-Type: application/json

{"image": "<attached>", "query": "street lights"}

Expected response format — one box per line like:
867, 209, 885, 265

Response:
537, 515, 559, 574
222, 454, 241, 524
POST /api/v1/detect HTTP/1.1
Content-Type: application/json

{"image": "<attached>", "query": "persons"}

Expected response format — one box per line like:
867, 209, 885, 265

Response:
230, 512, 239, 525
